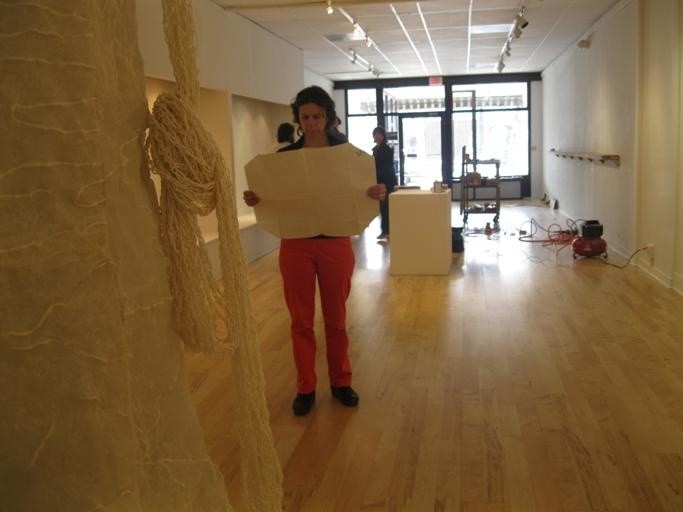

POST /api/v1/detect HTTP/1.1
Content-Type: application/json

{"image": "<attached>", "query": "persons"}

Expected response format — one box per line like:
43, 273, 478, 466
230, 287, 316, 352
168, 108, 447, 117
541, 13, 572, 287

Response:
272, 121, 296, 153
243, 86, 386, 416
372, 126, 397, 239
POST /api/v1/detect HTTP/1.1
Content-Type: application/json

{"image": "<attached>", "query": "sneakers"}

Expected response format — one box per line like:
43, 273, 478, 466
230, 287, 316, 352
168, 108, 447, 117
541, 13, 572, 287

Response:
376, 233, 390, 238
330, 382, 359, 406
292, 388, 315, 416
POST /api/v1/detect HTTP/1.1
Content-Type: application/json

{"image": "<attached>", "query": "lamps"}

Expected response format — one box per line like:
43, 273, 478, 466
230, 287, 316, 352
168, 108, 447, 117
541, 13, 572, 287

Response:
513, 14, 529, 30
